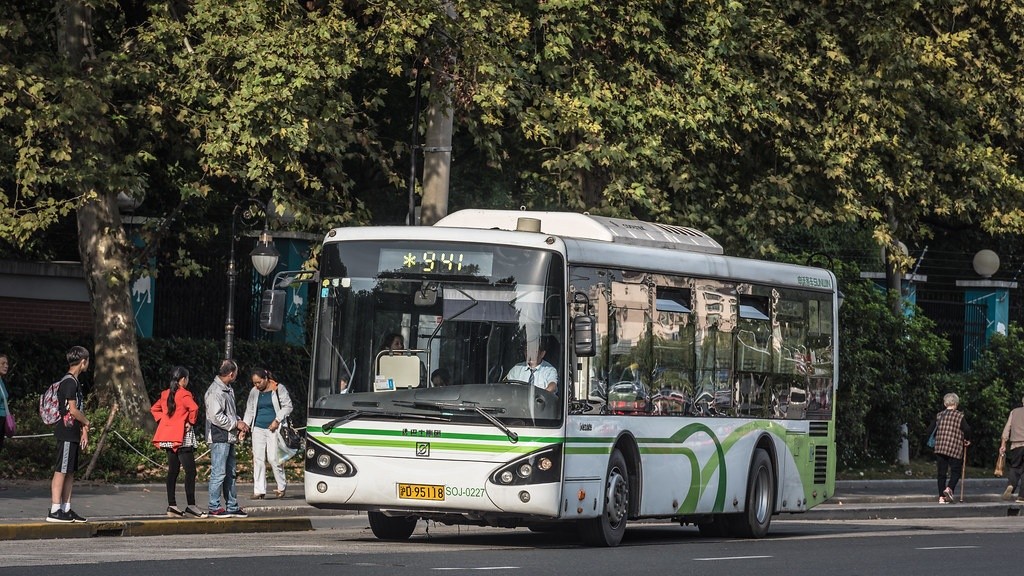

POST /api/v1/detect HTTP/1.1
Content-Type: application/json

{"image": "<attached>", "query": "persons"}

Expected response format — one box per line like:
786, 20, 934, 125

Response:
203, 359, 250, 518
380, 333, 405, 356
0, 354, 11, 446
431, 369, 453, 387
238, 367, 294, 501
151, 366, 209, 519
926, 392, 972, 505
501, 338, 558, 395
340, 375, 352, 394
47, 346, 91, 523
999, 396, 1024, 503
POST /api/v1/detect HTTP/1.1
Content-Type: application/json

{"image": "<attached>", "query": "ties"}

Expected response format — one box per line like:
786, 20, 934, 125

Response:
528, 368, 537, 385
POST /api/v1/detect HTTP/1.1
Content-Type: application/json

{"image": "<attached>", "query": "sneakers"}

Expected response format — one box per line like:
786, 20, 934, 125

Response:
209, 508, 231, 517
65, 509, 87, 522
1002, 486, 1024, 503
227, 510, 248, 518
939, 487, 954, 505
46, 508, 75, 523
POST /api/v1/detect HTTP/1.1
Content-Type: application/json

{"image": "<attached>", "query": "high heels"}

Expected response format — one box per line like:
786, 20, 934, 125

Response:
186, 507, 208, 519
167, 507, 186, 517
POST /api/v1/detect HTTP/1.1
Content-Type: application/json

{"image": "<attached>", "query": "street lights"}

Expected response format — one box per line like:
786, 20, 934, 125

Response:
224, 198, 281, 360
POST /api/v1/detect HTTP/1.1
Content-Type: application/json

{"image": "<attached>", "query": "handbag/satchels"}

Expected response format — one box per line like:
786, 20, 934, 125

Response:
275, 430, 298, 467
994, 453, 1006, 478
928, 437, 935, 447
6, 415, 15, 438
280, 426, 301, 449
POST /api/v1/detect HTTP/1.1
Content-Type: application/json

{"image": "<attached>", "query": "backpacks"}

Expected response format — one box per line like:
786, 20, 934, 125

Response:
39, 375, 78, 425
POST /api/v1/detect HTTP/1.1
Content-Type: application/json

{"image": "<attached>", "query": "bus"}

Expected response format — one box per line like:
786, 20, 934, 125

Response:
259, 205, 840, 547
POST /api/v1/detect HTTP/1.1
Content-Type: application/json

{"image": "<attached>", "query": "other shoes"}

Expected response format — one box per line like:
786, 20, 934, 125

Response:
250, 494, 265, 500
273, 489, 285, 499
